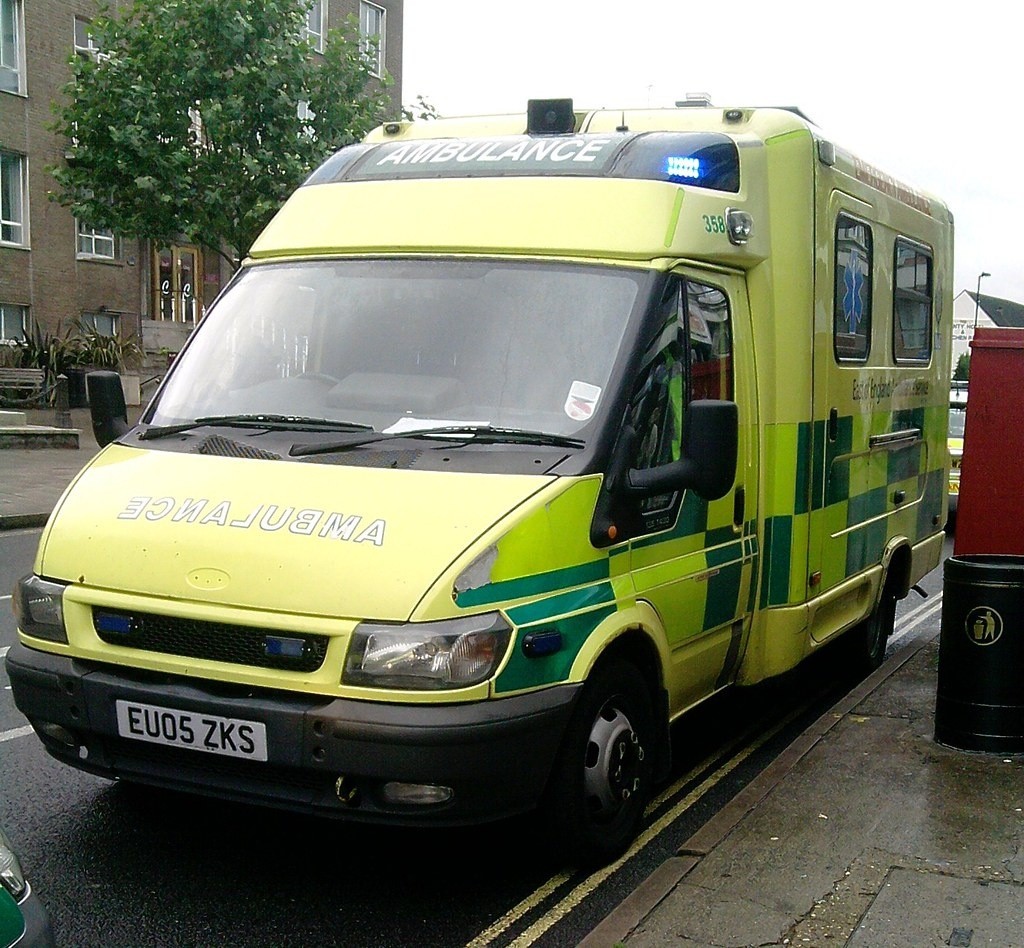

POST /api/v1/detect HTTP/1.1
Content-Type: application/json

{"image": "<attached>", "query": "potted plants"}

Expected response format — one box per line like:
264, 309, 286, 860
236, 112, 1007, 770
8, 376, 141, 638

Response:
0, 309, 147, 406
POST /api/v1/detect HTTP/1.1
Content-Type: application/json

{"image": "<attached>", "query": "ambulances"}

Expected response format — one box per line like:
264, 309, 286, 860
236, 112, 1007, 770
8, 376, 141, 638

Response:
4, 98, 956, 870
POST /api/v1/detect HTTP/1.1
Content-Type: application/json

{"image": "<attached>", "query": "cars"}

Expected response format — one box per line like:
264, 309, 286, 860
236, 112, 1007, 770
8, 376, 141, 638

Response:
1, 823, 57, 948
946, 379, 970, 511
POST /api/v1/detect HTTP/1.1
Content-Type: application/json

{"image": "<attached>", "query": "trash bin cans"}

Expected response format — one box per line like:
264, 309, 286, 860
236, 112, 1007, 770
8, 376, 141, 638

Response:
932, 554, 1024, 757
